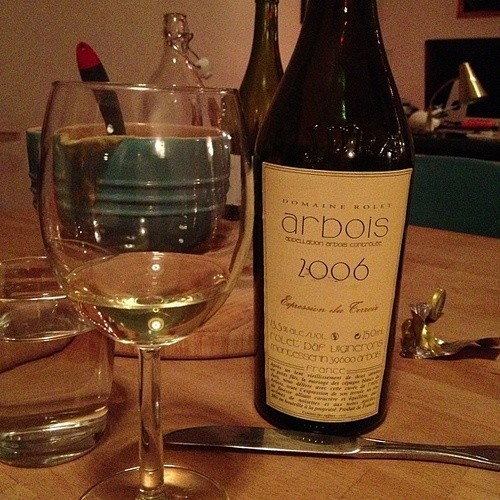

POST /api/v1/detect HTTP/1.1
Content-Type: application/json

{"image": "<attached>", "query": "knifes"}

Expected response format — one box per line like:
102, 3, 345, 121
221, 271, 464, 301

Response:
164, 424, 499, 471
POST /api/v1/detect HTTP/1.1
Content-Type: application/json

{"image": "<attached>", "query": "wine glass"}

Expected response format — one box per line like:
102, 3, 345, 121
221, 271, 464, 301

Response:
35, 78, 250, 500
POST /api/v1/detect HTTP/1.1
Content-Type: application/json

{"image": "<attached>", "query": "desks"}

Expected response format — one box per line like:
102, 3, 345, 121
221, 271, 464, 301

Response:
0, 144, 499, 500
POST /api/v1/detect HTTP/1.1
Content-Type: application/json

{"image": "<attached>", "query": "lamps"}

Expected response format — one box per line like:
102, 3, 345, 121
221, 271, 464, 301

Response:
421, 60, 485, 135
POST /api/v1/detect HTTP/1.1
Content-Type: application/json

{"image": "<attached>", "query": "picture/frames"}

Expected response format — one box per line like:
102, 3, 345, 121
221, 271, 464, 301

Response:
457, 0, 500, 19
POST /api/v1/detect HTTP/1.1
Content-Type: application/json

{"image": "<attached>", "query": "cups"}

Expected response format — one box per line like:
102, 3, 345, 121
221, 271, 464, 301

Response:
26, 126, 43, 203
1, 253, 116, 468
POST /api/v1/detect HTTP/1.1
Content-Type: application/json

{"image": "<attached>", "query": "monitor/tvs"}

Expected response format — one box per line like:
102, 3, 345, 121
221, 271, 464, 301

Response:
424, 37, 500, 128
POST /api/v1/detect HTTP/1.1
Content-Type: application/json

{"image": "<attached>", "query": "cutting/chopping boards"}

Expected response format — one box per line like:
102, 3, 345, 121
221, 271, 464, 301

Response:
114, 270, 254, 357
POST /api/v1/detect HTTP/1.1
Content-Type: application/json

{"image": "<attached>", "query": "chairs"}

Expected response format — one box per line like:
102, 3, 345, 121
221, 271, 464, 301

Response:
407, 155, 500, 238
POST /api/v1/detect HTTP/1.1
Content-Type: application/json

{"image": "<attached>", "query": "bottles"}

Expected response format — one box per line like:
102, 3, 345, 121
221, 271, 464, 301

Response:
253, 0, 414, 447
235, 1, 283, 148
143, 13, 206, 127
